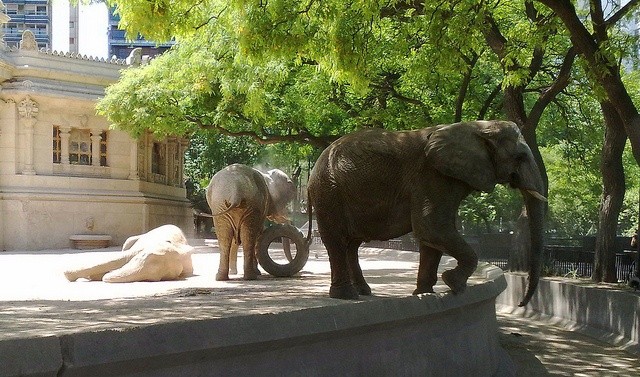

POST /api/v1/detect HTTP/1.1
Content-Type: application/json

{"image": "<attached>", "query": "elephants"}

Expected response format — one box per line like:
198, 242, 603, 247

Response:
191, 163, 298, 281
304, 121, 548, 307
66, 224, 193, 284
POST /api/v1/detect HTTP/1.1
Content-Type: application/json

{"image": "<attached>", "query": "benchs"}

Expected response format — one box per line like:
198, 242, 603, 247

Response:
68, 234, 112, 249
